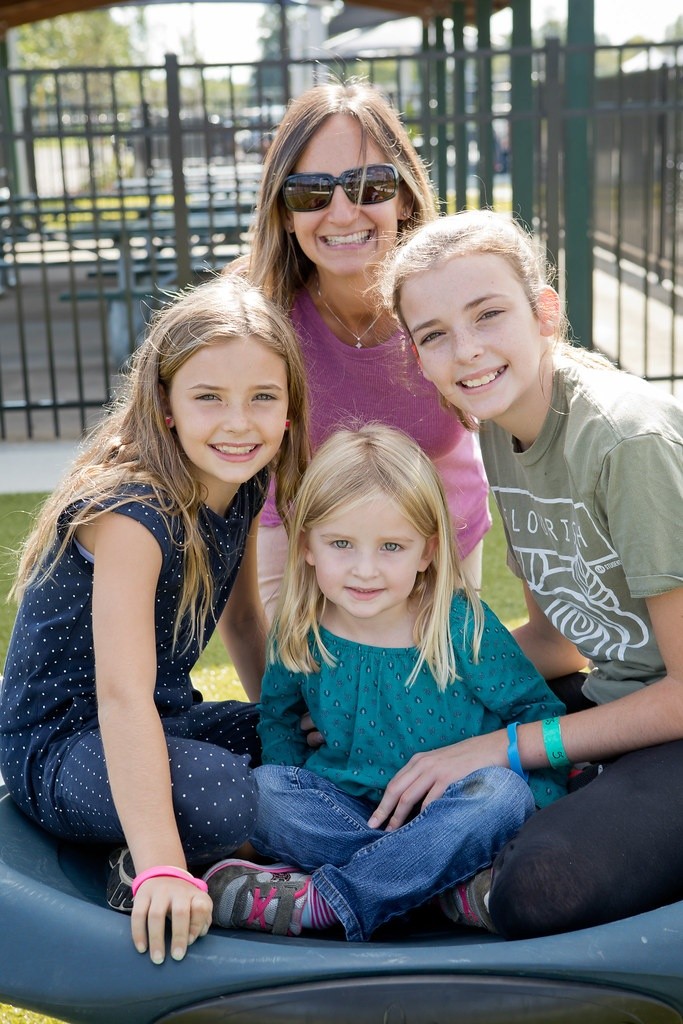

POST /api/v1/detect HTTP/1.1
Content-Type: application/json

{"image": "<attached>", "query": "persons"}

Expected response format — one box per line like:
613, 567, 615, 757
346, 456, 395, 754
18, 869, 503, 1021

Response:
204, 65, 495, 643
0, 269, 313, 965
301, 201, 683, 941
201, 409, 567, 938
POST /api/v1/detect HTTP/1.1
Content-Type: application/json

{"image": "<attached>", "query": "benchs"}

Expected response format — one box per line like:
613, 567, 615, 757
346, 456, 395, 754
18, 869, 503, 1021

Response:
0, 173, 278, 374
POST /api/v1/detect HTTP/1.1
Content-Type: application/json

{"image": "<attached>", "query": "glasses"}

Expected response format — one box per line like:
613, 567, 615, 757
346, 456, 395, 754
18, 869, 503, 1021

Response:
282, 163, 403, 213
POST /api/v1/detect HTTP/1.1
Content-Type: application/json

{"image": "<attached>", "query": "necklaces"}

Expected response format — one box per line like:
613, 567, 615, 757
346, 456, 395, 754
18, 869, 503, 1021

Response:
313, 269, 388, 350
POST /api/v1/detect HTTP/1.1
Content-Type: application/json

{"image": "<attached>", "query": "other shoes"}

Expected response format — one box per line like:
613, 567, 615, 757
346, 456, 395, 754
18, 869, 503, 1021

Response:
104, 846, 138, 912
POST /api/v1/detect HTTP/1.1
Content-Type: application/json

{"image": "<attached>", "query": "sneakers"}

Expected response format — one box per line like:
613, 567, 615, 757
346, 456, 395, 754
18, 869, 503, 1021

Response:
201, 858, 313, 938
442, 868, 501, 936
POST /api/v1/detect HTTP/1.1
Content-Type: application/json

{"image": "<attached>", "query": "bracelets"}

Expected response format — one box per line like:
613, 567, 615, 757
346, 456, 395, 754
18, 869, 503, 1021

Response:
131, 867, 209, 895
505, 719, 529, 783
541, 715, 573, 777
